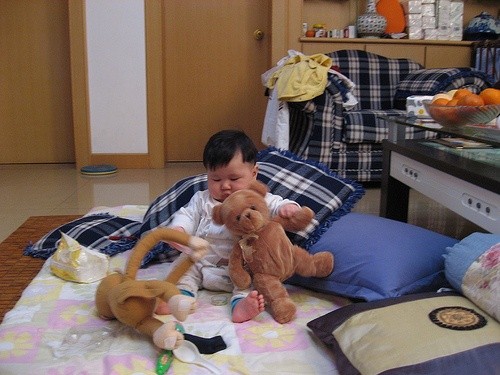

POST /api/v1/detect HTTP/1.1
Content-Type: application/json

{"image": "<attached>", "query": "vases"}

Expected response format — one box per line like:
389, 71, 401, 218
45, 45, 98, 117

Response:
355, 0, 387, 39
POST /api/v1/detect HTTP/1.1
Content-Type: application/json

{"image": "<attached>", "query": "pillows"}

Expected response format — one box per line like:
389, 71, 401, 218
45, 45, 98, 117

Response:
139, 173, 209, 269
440, 231, 500, 323
305, 290, 500, 375
23, 212, 143, 260
282, 210, 460, 302
256, 143, 367, 250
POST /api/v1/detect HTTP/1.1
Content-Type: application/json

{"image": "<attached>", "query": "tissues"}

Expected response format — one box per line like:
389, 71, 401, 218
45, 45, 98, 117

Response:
49, 231, 111, 284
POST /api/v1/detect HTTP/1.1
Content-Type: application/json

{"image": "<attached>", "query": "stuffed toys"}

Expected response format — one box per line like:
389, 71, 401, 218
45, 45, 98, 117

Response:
212, 179, 334, 324
95, 227, 210, 350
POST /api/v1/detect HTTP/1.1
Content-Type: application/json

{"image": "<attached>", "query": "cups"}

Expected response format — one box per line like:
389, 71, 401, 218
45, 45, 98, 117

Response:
315, 29, 325, 37
326, 26, 356, 38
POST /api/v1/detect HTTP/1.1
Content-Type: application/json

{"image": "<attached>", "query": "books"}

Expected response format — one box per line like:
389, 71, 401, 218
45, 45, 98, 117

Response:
431, 138, 493, 149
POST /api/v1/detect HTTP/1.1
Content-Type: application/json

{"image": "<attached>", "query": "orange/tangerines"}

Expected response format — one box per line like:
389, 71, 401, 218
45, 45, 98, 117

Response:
431, 88, 500, 127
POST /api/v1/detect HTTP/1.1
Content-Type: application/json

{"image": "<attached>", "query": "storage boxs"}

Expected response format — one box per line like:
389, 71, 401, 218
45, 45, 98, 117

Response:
406, 94, 435, 119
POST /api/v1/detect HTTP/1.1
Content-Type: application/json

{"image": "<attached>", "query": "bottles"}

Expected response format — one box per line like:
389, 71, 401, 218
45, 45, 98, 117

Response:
357, 0, 387, 39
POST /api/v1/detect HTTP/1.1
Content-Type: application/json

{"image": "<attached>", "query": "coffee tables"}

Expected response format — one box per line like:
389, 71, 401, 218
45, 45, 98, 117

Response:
374, 112, 500, 235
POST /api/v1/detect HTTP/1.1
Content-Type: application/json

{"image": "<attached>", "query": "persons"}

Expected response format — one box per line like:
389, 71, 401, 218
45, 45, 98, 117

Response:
154, 130, 301, 323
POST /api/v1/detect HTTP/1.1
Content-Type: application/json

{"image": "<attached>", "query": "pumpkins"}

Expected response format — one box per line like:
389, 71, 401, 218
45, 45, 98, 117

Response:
375, 0, 405, 34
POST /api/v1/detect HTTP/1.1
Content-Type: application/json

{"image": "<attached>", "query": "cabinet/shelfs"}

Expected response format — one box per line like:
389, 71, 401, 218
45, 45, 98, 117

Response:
288, 0, 500, 68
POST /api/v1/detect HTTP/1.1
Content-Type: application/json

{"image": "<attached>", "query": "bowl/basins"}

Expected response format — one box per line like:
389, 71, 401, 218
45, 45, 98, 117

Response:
422, 99, 500, 130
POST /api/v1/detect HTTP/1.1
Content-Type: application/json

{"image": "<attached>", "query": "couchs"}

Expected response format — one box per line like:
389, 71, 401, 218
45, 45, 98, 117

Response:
286, 49, 497, 189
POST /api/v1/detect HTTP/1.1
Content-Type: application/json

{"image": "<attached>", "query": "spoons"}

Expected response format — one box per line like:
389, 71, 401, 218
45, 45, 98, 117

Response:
172, 340, 221, 375
